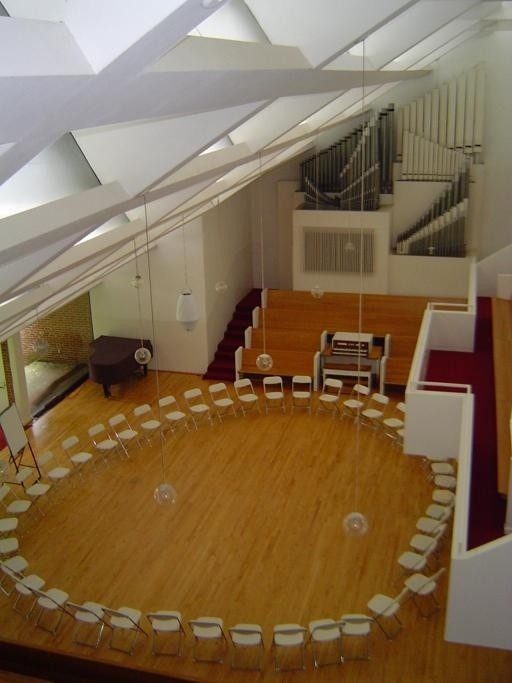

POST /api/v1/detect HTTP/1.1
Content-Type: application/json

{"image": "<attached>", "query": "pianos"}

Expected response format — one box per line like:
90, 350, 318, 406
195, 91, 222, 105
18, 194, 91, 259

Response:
88, 334, 153, 396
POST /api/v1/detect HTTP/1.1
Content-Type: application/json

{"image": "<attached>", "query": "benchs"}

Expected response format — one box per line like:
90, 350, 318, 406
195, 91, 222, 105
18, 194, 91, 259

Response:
236, 290, 468, 385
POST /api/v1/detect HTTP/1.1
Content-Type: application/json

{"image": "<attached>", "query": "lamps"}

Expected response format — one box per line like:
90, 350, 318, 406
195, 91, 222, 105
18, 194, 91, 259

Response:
345, 41, 369, 538
344, 136, 356, 253
133, 239, 151, 366
175, 211, 200, 332
256, 155, 274, 373
310, 149, 324, 298
142, 195, 179, 507
215, 200, 228, 294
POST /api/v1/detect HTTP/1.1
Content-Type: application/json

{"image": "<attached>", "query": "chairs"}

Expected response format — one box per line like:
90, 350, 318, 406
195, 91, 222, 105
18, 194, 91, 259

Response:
0, 375, 458, 671
